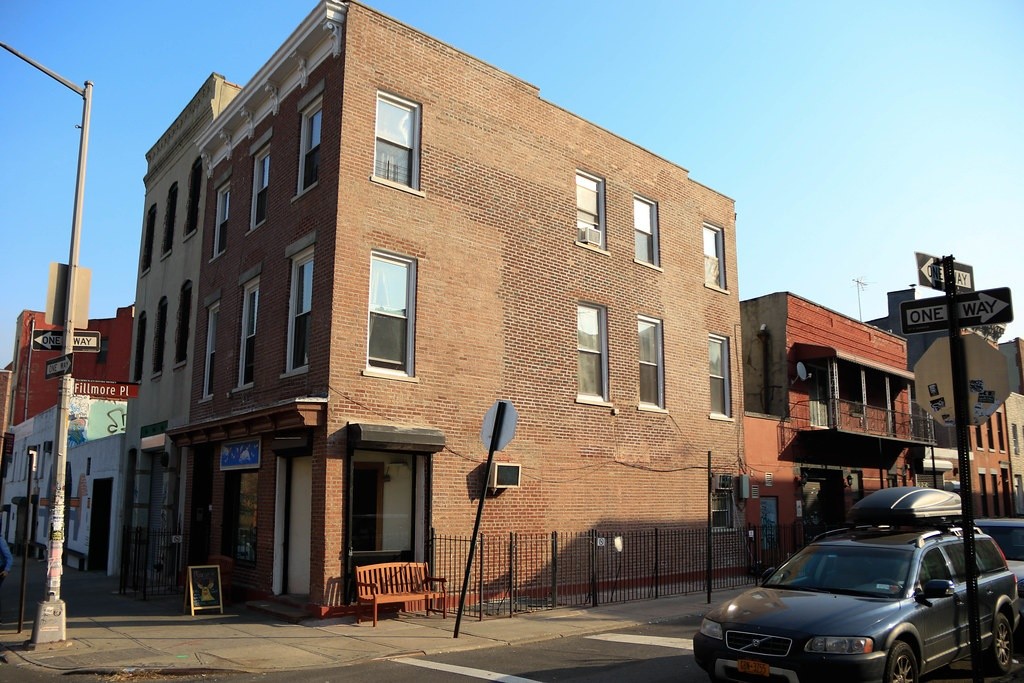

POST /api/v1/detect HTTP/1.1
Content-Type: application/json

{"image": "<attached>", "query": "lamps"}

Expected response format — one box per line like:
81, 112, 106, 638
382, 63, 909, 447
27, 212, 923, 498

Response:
795, 471, 808, 487
843, 476, 852, 488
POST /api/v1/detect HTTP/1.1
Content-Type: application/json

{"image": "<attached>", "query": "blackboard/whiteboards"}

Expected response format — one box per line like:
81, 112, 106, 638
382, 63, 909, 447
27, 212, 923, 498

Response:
184, 565, 223, 610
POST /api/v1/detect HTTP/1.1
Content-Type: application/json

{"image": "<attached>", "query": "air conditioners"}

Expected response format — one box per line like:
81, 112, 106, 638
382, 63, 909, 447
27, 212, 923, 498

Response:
580, 227, 601, 245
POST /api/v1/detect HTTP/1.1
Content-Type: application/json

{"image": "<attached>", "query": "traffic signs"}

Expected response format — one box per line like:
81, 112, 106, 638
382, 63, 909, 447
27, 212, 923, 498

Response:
918, 253, 975, 294
44, 353, 74, 381
31, 329, 101, 353
899, 287, 1013, 335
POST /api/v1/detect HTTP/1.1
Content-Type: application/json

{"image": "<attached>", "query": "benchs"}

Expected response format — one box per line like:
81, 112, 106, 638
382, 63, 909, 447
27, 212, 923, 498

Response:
203, 556, 235, 594
355, 562, 447, 627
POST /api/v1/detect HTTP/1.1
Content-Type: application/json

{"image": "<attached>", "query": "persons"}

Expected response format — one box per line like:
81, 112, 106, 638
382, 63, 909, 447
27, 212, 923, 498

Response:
0, 535, 12, 623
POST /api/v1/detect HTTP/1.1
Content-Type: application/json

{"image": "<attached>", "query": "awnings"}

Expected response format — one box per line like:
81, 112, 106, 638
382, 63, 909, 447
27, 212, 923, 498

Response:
793, 342, 915, 442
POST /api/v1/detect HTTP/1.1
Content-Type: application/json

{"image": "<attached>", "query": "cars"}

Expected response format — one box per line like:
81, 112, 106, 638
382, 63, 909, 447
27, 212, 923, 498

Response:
692, 488, 1019, 683
947, 518, 1024, 624
